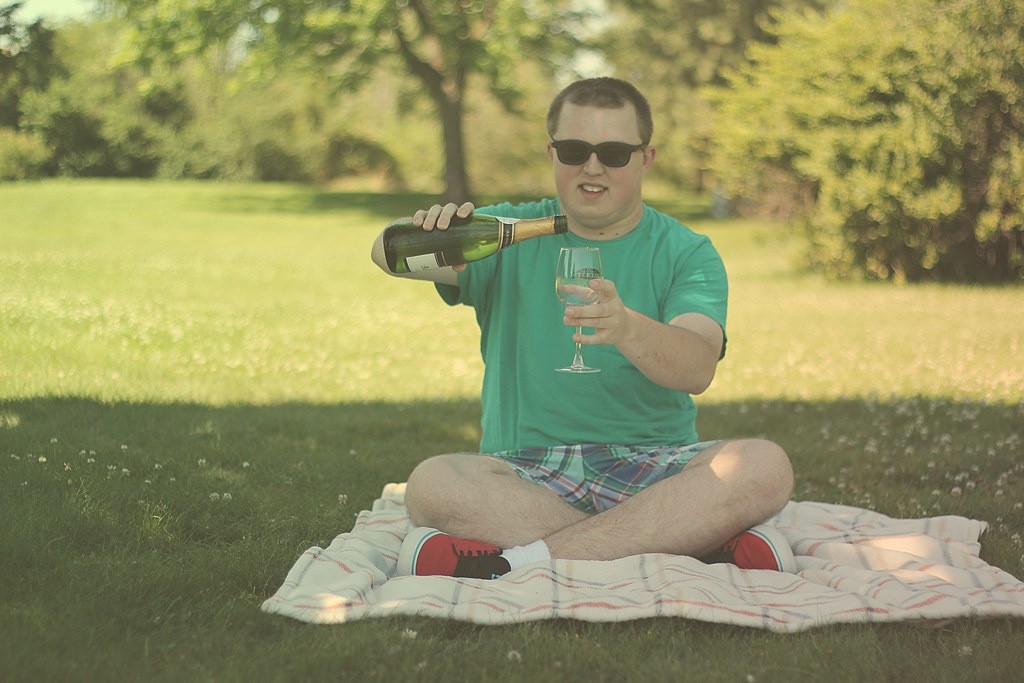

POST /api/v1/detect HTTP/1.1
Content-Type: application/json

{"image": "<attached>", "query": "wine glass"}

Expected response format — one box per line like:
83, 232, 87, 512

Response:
554, 246, 605, 375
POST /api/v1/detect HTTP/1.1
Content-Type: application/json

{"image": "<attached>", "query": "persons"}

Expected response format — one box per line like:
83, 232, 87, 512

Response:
372, 77, 798, 578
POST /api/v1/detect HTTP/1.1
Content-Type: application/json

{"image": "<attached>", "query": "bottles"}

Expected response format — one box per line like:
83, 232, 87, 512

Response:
382, 212, 568, 274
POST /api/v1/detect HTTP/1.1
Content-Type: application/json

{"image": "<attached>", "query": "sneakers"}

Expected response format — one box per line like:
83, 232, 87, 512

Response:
700, 525, 797, 575
396, 527, 511, 580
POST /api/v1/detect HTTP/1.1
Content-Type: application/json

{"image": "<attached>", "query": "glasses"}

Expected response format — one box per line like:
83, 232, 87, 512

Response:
550, 139, 646, 167
